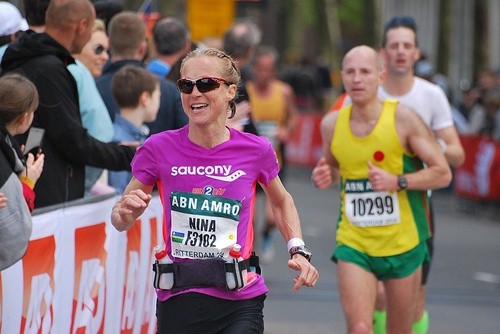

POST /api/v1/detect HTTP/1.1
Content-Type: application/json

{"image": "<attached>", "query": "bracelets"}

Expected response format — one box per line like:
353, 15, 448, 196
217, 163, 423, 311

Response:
287, 238, 305, 253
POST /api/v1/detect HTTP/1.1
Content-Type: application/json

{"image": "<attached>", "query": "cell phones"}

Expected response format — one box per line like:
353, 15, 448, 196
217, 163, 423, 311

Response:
22, 127, 45, 161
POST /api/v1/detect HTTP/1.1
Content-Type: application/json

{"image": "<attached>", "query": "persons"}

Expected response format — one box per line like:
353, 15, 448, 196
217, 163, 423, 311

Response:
94, 12, 188, 139
0, 2, 30, 49
312, 45, 452, 334
111, 46, 319, 334
342, 15, 465, 334
415, 55, 500, 140
0, 76, 45, 273
0, 0, 113, 197
145, 18, 346, 188
0, 0, 142, 209
108, 65, 161, 194
67, 19, 114, 196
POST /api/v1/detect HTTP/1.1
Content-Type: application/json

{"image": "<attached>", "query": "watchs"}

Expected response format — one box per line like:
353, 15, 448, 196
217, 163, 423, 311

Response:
397, 174, 408, 192
289, 244, 312, 262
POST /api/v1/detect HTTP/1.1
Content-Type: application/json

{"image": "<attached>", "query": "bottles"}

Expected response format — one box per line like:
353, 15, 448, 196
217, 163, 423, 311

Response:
154, 246, 174, 290
225, 244, 247, 289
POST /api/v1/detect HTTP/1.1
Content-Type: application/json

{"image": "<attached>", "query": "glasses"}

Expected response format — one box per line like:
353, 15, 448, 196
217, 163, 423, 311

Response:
177, 77, 229, 94
89, 45, 111, 55
